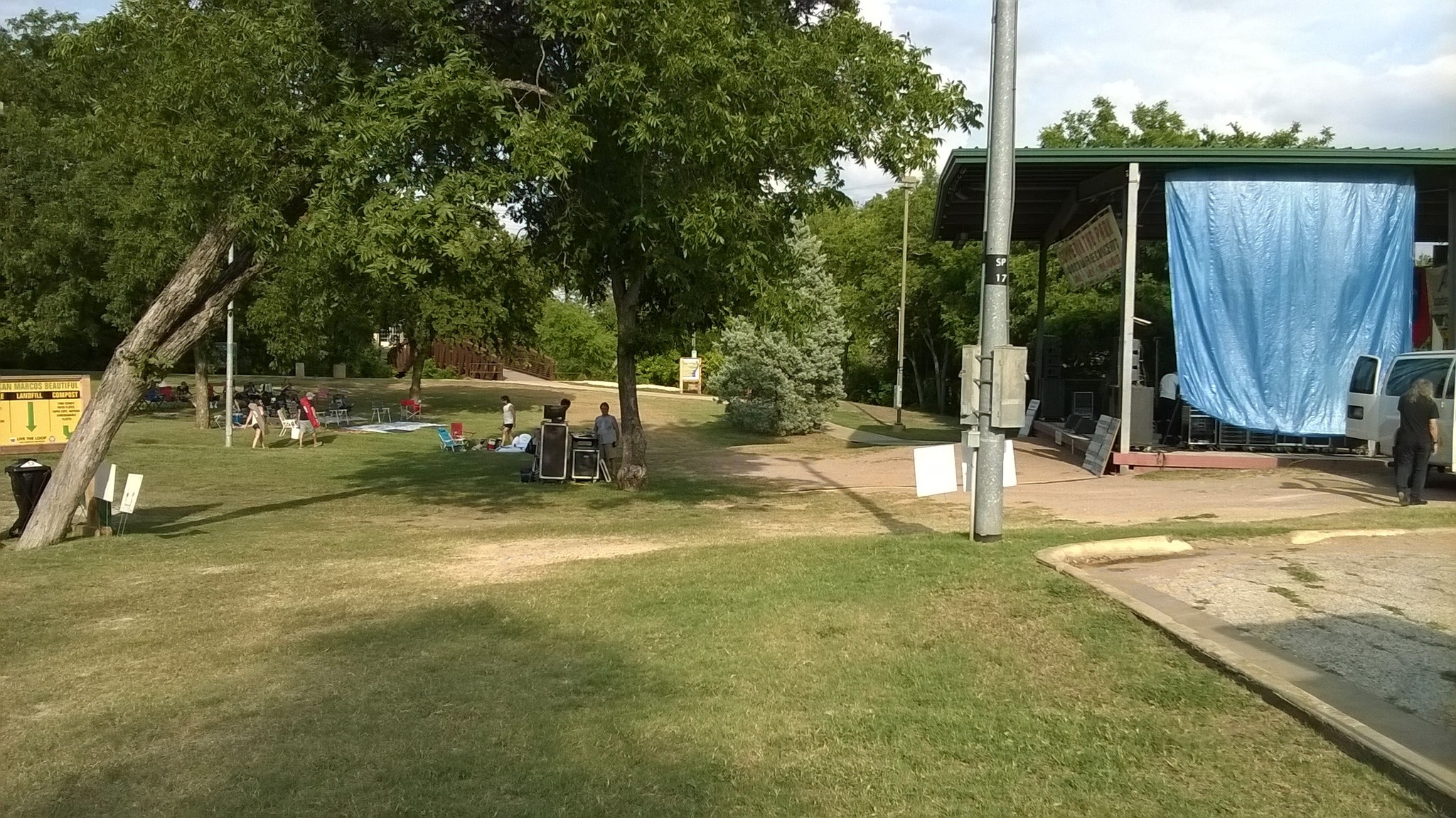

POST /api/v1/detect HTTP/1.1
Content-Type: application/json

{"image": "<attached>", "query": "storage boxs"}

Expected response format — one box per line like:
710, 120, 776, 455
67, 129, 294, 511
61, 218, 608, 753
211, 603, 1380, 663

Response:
570, 449, 601, 480
540, 422, 569, 479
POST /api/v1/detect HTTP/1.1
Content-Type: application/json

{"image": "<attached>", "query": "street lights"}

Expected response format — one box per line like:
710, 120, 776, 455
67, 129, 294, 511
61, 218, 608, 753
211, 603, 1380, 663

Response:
890, 176, 920, 432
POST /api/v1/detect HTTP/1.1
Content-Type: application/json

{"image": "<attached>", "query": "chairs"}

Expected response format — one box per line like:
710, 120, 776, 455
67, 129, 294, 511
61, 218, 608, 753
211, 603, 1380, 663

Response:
437, 422, 476, 451
399, 399, 422, 421
132, 386, 354, 437
371, 401, 392, 423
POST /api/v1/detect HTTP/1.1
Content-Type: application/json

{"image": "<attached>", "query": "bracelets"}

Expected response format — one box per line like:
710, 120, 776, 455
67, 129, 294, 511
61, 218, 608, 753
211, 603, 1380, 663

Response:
1434, 442, 1437, 443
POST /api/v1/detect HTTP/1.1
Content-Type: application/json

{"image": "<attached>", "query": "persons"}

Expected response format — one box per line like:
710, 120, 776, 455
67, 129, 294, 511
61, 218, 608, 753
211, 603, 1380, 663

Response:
593, 402, 620, 478
1394, 378, 1440, 506
244, 398, 265, 448
208, 383, 220, 409
298, 392, 322, 449
177, 382, 197, 408
552, 399, 570, 423
501, 396, 516, 447
245, 382, 261, 400
1159, 366, 1179, 420
282, 378, 292, 392
223, 380, 250, 413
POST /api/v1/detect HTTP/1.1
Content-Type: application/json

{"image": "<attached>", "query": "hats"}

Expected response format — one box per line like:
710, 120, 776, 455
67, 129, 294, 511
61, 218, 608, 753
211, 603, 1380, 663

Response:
306, 392, 316, 397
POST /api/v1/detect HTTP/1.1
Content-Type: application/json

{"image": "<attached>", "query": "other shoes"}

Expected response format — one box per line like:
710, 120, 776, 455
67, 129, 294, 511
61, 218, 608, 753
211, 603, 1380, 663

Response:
214, 405, 218, 409
210, 405, 213, 409
236, 410, 242, 413
313, 441, 322, 448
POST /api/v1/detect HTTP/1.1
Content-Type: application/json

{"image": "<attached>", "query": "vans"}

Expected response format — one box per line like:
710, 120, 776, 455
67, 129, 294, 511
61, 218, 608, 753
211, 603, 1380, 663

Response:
1345, 350, 1456, 480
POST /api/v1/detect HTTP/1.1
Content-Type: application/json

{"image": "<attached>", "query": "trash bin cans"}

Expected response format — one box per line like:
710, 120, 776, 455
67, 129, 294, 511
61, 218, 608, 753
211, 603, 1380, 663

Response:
4, 458, 52, 538
296, 362, 304, 378
333, 363, 346, 378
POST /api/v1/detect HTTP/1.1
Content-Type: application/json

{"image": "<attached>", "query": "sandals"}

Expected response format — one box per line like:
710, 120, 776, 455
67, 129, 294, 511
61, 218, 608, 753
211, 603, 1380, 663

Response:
611, 474, 616, 481
